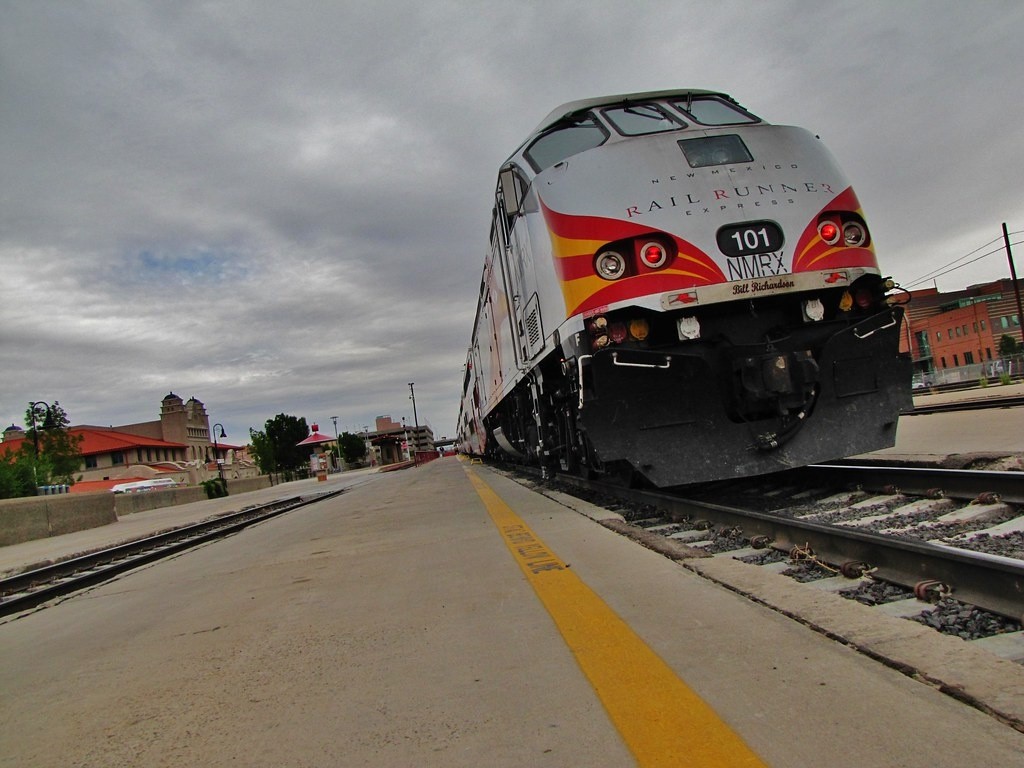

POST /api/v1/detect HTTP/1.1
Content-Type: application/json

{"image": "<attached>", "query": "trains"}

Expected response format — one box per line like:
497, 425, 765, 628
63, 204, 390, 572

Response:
452, 89, 915, 488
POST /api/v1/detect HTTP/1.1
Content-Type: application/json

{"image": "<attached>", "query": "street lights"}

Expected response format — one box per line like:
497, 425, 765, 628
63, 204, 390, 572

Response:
407, 382, 422, 451
213, 423, 229, 496
31, 402, 61, 537
330, 416, 343, 473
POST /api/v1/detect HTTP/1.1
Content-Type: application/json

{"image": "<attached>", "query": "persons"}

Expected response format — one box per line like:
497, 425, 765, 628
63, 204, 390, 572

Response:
372, 458, 376, 467
439, 446, 445, 457
454, 445, 459, 455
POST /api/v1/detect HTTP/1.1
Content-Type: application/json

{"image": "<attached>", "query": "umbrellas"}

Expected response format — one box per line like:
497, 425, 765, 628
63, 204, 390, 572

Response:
296, 433, 338, 448
